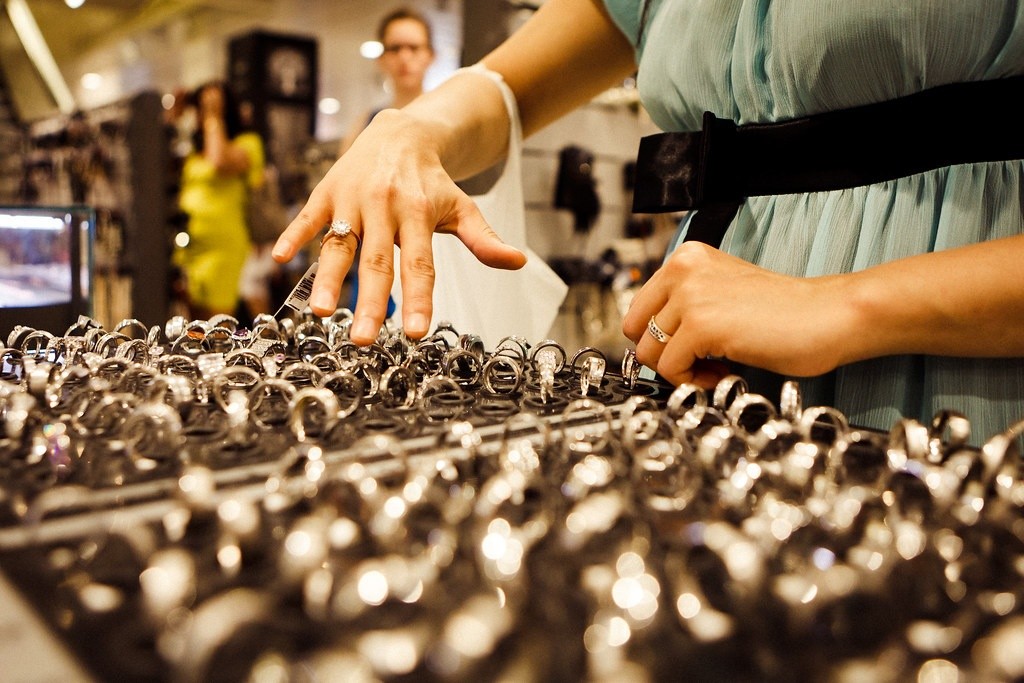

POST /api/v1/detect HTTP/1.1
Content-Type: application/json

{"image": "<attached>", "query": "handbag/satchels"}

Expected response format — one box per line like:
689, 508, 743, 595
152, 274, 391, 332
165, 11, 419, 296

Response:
387, 63, 567, 344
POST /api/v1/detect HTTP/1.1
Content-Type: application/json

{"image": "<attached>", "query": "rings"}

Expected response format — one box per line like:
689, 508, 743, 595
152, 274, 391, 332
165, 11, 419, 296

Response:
323, 220, 361, 248
0, 304, 1024, 683
648, 316, 671, 343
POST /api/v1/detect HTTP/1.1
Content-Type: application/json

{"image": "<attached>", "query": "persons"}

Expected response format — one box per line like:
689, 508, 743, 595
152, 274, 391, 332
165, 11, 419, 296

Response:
273, 0, 1024, 392
369, 7, 435, 125
173, 82, 268, 322
